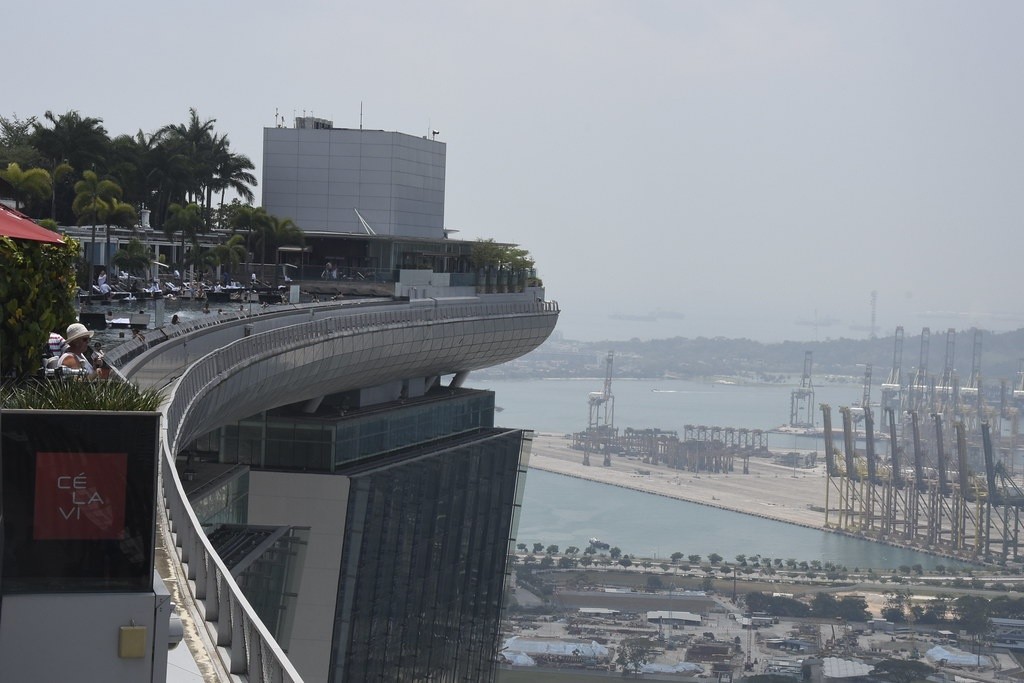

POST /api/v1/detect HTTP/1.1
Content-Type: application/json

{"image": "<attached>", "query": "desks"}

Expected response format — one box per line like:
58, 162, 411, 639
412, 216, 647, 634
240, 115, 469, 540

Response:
222, 288, 246, 298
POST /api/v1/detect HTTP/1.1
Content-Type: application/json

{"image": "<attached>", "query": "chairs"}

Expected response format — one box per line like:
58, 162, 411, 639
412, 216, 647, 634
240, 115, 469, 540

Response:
93, 285, 151, 298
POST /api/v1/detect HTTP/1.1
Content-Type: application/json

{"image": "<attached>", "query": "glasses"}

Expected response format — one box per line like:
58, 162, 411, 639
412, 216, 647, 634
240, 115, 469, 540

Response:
78, 336, 91, 343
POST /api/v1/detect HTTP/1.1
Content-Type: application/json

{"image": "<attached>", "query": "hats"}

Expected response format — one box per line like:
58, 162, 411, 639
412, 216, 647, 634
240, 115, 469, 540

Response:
63, 323, 94, 344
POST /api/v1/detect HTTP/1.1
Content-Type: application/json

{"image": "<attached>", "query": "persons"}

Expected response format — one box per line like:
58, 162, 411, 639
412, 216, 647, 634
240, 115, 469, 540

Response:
313, 295, 319, 302
261, 301, 269, 308
238, 305, 244, 310
150, 283, 159, 291
337, 292, 344, 300
107, 310, 113, 321
203, 303, 211, 313
281, 292, 286, 304
98, 270, 111, 301
139, 310, 144, 314
223, 271, 229, 284
118, 332, 124, 338
172, 315, 180, 325
250, 270, 257, 285
171, 293, 177, 301
42, 322, 106, 382
218, 309, 222, 314
197, 287, 206, 298
173, 269, 180, 281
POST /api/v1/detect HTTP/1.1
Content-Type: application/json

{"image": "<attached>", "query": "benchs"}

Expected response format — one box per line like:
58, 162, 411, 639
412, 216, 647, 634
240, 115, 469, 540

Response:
206, 292, 223, 303
259, 294, 283, 305
